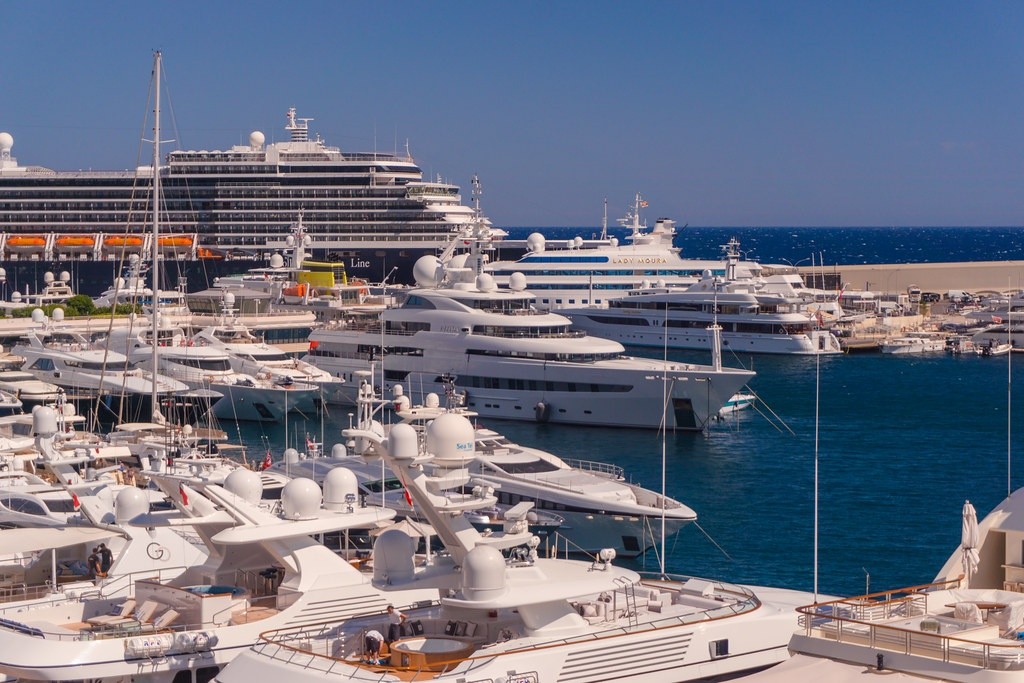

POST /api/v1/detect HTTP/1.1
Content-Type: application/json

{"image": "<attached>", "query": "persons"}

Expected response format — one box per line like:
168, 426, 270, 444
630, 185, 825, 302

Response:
96, 543, 114, 577
387, 605, 408, 644
87, 548, 102, 579
365, 630, 385, 666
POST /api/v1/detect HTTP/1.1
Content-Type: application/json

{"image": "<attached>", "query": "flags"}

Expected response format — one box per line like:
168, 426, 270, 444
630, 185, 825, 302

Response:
305, 436, 314, 450
71, 491, 81, 511
839, 284, 848, 303
180, 483, 188, 506
992, 315, 1002, 323
815, 307, 824, 327
640, 200, 648, 208
261, 448, 272, 471
401, 474, 413, 506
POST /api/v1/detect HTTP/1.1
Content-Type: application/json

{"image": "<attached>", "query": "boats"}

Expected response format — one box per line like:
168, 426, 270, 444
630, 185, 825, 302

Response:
0, 49, 1024, 683
